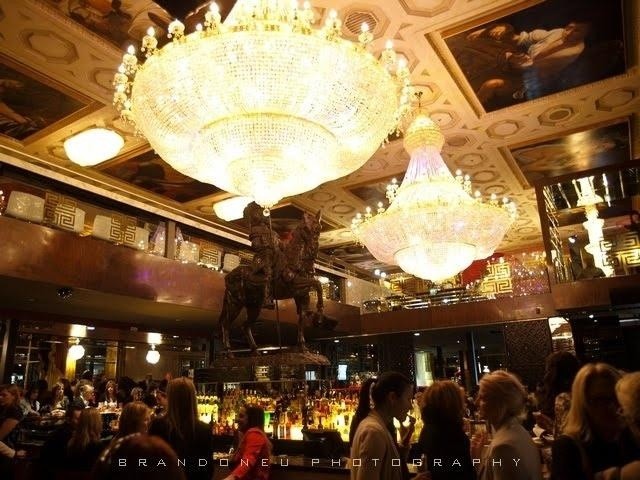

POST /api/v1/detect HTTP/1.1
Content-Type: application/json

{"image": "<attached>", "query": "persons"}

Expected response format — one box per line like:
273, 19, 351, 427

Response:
244, 201, 282, 310
220, 403, 272, 480
551, 362, 640, 480
349, 370, 431, 480
0, 374, 213, 480
474, 369, 542, 480
464, 14, 592, 112
407, 380, 478, 480
614, 371, 640, 480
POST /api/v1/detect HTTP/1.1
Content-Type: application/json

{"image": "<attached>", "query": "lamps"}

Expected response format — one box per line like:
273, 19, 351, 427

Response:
113, 0, 414, 216
350, 92, 518, 286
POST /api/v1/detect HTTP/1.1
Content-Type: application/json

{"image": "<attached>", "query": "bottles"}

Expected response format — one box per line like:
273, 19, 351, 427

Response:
218, 387, 257, 414
211, 415, 236, 435
264, 415, 291, 438
257, 382, 307, 414
302, 415, 351, 430
307, 382, 359, 416
195, 390, 218, 414
